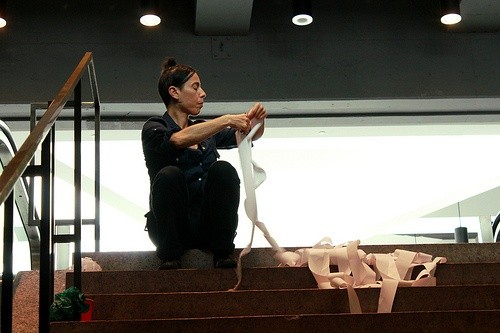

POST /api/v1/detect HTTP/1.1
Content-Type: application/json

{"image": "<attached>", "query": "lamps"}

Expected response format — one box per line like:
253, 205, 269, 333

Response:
0, 0, 8, 28
291, 0, 313, 26
138, 0, 161, 27
438, 0, 462, 25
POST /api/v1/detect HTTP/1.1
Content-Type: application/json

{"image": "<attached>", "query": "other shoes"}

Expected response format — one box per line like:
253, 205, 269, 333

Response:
156, 256, 183, 270
214, 253, 237, 269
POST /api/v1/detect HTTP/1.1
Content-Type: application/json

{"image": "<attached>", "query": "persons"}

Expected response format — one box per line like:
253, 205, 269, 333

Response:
142, 58, 268, 272
